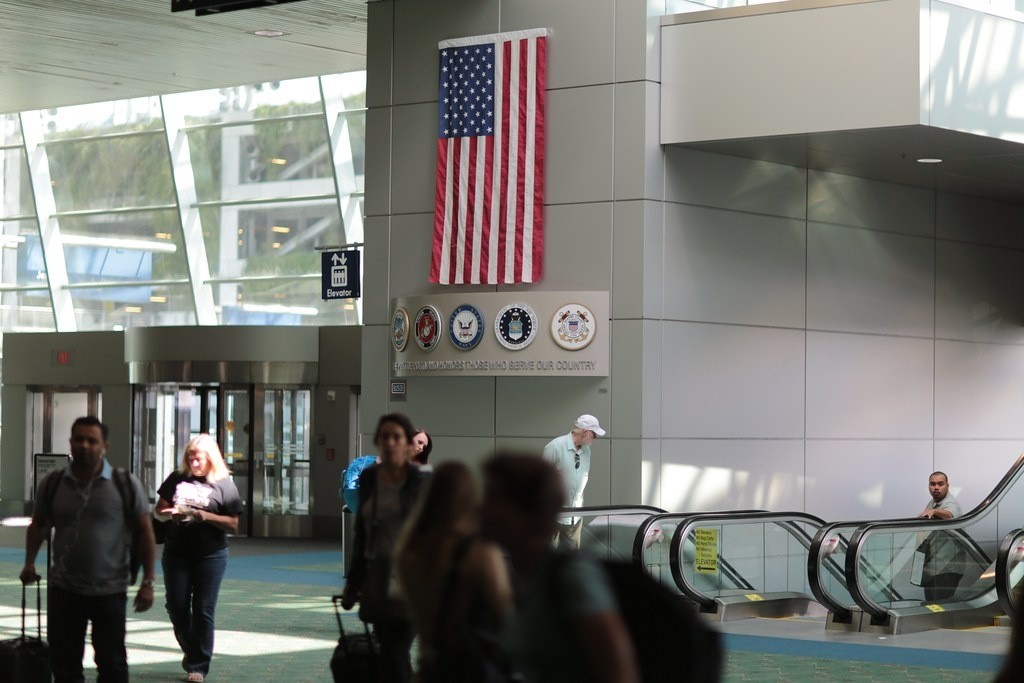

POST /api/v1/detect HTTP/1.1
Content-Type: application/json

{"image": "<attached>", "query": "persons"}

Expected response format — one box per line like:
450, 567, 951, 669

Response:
19, 417, 155, 683
542, 414, 606, 549
342, 412, 639, 683
916, 472, 965, 600
154, 434, 244, 683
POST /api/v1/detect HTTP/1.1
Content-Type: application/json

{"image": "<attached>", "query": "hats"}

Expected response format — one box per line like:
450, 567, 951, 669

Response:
575, 414, 606, 437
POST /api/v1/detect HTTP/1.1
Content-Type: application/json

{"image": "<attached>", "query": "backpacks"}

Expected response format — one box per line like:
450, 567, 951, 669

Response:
341, 454, 376, 515
544, 557, 721, 683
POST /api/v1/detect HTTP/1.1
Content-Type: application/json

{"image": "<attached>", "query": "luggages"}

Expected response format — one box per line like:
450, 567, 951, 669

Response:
0, 573, 51, 683
330, 594, 385, 683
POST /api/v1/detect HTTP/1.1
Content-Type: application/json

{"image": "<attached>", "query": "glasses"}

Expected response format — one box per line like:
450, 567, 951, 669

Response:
574, 454, 581, 469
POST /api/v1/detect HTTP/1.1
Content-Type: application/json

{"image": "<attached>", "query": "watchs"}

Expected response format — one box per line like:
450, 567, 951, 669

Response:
141, 580, 153, 587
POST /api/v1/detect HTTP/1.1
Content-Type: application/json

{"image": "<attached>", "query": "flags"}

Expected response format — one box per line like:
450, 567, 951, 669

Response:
428, 28, 548, 285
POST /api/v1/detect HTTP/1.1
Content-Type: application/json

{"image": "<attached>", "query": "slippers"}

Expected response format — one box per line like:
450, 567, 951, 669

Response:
187, 670, 203, 682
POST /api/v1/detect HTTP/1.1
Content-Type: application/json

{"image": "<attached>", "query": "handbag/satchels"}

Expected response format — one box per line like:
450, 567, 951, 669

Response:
910, 540, 930, 587
407, 544, 515, 683
